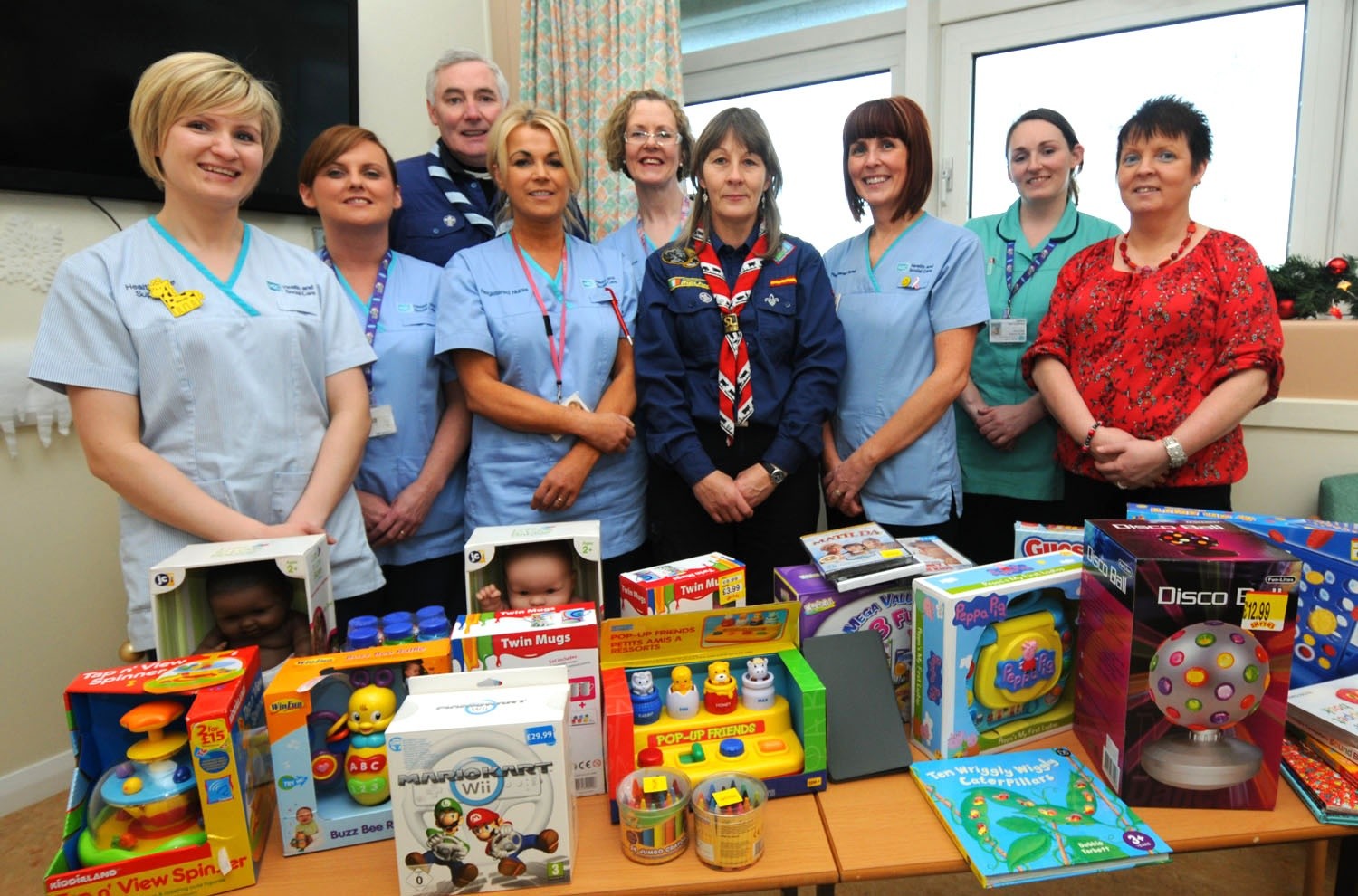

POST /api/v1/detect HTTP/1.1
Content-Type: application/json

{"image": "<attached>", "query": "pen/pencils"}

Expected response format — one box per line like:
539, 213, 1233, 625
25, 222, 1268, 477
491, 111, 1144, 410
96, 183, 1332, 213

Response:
604, 287, 633, 346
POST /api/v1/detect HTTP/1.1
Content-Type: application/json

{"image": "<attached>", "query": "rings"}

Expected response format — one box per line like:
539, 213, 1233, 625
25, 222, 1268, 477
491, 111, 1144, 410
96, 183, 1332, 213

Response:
834, 489, 841, 496
558, 497, 563, 502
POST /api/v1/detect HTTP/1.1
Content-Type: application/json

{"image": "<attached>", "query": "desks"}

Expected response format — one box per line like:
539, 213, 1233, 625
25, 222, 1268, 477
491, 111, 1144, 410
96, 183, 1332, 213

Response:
814, 729, 1358, 896
213, 792, 839, 896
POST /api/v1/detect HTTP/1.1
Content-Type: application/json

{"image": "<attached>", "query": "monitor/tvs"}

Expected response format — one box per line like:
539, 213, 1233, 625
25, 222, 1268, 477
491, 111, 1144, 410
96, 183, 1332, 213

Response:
0, 1, 361, 215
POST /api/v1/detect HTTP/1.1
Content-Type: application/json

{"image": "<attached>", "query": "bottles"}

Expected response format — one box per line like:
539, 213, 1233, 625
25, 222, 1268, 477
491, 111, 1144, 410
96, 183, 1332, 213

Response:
341, 605, 451, 651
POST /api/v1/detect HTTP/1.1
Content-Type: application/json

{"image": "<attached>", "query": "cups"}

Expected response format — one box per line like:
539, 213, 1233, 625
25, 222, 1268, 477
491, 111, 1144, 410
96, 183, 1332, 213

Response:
616, 765, 692, 866
689, 774, 770, 871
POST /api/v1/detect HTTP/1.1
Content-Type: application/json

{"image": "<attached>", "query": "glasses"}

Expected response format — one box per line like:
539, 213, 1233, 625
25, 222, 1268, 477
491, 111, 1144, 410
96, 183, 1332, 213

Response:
623, 130, 684, 147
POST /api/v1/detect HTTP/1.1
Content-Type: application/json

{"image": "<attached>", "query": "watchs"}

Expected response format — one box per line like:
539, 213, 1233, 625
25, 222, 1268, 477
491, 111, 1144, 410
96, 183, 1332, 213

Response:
759, 460, 785, 484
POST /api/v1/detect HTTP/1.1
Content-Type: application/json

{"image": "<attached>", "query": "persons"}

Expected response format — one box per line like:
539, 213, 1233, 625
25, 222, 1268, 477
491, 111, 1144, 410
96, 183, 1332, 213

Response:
28, 52, 386, 666
191, 560, 311, 670
1022, 94, 1285, 528
299, 124, 471, 616
475, 540, 585, 612
633, 107, 846, 604
593, 87, 696, 290
434, 102, 648, 621
820, 94, 991, 552
952, 108, 1124, 563
386, 48, 591, 267
821, 538, 901, 560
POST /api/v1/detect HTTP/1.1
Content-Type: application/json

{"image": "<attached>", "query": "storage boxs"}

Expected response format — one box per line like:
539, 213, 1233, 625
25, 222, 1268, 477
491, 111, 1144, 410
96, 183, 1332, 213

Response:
263, 638, 453, 856
617, 552, 746, 618
909, 548, 1086, 758
450, 601, 606, 799
1073, 518, 1301, 816
385, 664, 578, 896
1125, 503, 1358, 687
600, 600, 828, 829
42, 645, 277, 896
773, 536, 972, 721
1014, 523, 1085, 557
150, 534, 336, 683
465, 520, 604, 621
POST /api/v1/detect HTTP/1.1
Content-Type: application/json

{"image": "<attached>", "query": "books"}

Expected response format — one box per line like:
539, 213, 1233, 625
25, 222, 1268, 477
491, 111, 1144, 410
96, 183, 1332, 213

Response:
1280, 675, 1358, 830
910, 746, 1173, 890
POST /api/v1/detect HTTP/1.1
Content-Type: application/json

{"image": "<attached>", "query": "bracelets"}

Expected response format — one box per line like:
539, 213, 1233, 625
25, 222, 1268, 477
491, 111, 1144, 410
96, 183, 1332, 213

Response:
1163, 435, 1187, 467
1082, 421, 1104, 452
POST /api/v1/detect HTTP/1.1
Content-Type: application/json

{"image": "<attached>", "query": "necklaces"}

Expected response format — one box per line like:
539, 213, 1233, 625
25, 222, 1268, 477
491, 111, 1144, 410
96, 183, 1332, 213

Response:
1119, 221, 1196, 272
